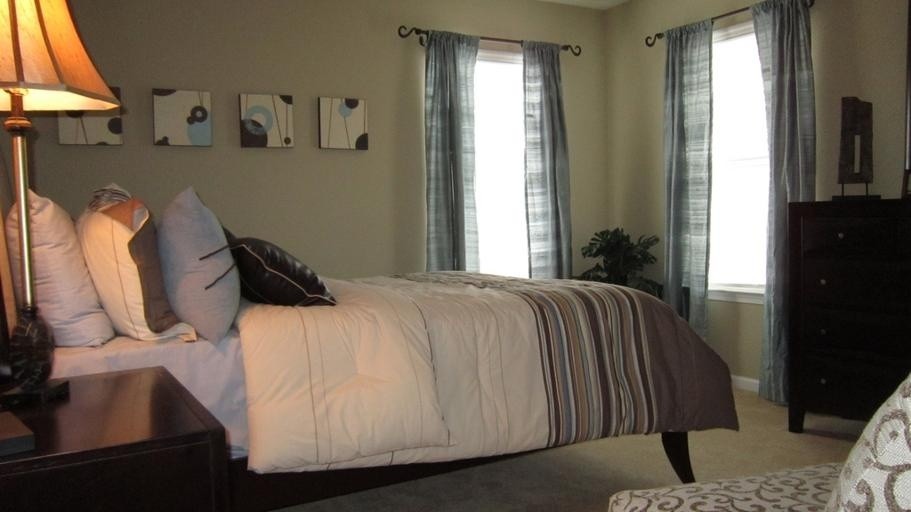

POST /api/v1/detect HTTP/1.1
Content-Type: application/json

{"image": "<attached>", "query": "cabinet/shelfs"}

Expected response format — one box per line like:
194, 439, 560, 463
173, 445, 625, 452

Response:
785, 200, 911, 435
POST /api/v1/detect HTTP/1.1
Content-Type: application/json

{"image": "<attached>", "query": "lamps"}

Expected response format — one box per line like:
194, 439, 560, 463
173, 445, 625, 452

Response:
0, 1, 125, 412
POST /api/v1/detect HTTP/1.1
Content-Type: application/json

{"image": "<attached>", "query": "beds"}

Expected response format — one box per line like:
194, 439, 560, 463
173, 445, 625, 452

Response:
2, 194, 716, 510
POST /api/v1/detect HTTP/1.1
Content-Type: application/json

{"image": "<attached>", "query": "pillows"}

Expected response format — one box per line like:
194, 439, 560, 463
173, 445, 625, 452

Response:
1, 186, 116, 344
157, 186, 239, 345
76, 184, 199, 342
219, 224, 337, 307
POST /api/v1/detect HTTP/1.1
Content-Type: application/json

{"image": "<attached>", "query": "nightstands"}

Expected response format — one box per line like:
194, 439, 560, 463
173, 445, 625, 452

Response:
0, 367, 229, 507
